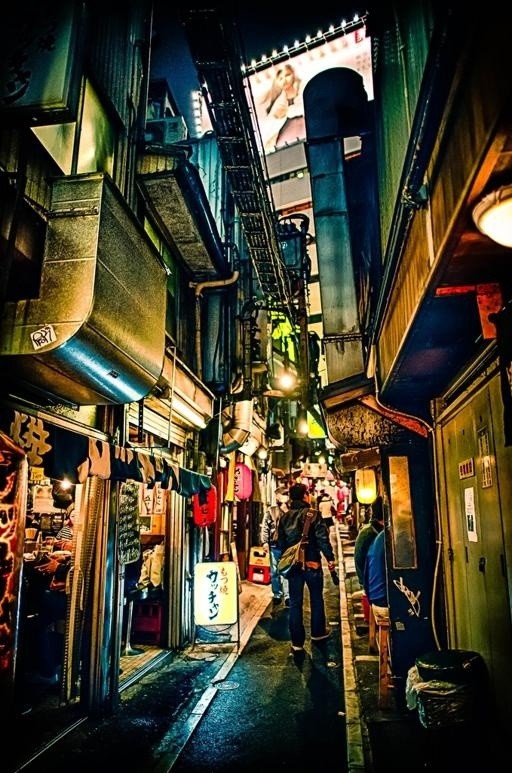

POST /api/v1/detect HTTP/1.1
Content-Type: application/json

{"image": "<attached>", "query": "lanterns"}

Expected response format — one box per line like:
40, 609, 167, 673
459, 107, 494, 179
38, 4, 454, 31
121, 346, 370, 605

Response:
356, 469, 377, 503
234, 463, 252, 500
193, 482, 218, 528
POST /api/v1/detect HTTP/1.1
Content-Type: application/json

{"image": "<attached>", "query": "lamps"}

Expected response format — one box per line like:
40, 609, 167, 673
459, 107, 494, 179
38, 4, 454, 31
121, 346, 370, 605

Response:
471, 185, 512, 249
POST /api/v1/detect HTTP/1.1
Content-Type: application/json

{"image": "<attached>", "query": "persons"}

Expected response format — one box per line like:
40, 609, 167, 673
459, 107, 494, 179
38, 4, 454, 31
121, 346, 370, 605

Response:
53, 502, 74, 583
261, 478, 389, 654
26, 535, 73, 693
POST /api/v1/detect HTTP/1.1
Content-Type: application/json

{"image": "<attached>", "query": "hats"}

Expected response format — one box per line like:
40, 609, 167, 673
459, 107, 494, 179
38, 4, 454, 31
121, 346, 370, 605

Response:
274, 485, 289, 495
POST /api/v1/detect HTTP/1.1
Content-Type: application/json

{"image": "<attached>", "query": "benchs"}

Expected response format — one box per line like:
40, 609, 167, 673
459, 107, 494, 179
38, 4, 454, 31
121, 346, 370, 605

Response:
368, 603, 394, 710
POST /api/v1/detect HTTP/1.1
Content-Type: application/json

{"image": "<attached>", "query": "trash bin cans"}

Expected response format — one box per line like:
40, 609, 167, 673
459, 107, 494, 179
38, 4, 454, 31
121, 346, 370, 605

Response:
415, 649, 491, 773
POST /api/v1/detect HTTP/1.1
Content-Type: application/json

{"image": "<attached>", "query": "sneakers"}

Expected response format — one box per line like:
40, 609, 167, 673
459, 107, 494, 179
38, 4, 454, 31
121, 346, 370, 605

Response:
272, 597, 290, 606
290, 639, 304, 651
310, 625, 332, 640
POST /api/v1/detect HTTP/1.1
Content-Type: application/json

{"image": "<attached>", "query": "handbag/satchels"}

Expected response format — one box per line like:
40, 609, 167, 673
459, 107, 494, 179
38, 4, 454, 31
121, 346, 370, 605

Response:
277, 542, 306, 579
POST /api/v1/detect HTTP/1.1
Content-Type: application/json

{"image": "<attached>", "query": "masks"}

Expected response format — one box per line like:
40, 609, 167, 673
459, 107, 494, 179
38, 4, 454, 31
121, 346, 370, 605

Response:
279, 495, 289, 503
